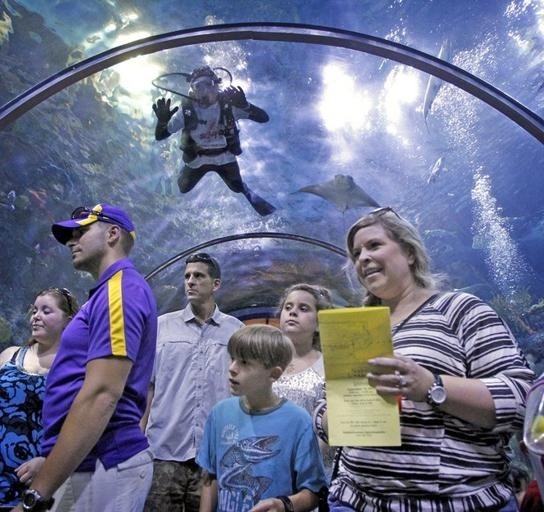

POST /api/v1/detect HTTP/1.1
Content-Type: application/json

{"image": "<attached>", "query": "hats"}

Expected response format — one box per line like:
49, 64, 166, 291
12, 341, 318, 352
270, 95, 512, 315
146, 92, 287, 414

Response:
52, 203, 136, 244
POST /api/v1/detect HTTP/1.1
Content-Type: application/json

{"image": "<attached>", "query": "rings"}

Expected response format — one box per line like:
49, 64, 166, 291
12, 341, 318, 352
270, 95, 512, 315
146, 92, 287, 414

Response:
29, 477, 34, 482
399, 375, 406, 387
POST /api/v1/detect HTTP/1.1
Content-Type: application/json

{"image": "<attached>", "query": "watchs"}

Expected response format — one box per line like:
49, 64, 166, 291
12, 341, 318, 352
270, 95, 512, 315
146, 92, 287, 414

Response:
21, 489, 54, 511
425, 373, 448, 410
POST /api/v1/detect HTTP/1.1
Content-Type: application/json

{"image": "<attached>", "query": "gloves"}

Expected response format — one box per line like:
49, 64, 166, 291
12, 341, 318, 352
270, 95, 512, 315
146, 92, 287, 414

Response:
153, 98, 178, 122
224, 85, 248, 108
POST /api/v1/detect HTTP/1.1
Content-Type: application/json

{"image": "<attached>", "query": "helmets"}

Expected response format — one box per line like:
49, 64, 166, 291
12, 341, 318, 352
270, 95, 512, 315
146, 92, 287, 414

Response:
190, 66, 220, 83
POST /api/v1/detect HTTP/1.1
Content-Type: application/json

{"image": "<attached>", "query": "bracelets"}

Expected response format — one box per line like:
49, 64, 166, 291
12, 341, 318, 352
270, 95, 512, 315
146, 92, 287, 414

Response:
278, 495, 294, 511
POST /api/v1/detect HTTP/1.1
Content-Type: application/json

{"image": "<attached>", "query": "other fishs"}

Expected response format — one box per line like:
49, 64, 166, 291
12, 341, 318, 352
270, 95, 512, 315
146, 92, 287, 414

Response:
290, 173, 380, 236
423, 45, 448, 136
454, 284, 487, 293
424, 229, 457, 240
427, 155, 447, 185
97, 0, 125, 31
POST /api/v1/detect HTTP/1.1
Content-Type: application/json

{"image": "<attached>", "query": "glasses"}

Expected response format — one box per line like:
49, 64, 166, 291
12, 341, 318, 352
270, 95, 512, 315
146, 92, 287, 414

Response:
186, 253, 215, 267
71, 207, 124, 225
58, 288, 72, 311
370, 206, 401, 220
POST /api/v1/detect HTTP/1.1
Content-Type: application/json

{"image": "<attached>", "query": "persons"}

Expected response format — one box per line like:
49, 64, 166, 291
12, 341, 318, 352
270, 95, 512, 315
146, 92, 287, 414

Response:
266, 282, 337, 479
195, 323, 328, 512
153, 67, 269, 196
0, 286, 81, 512
141, 253, 248, 512
9, 204, 156, 511
310, 206, 536, 512
